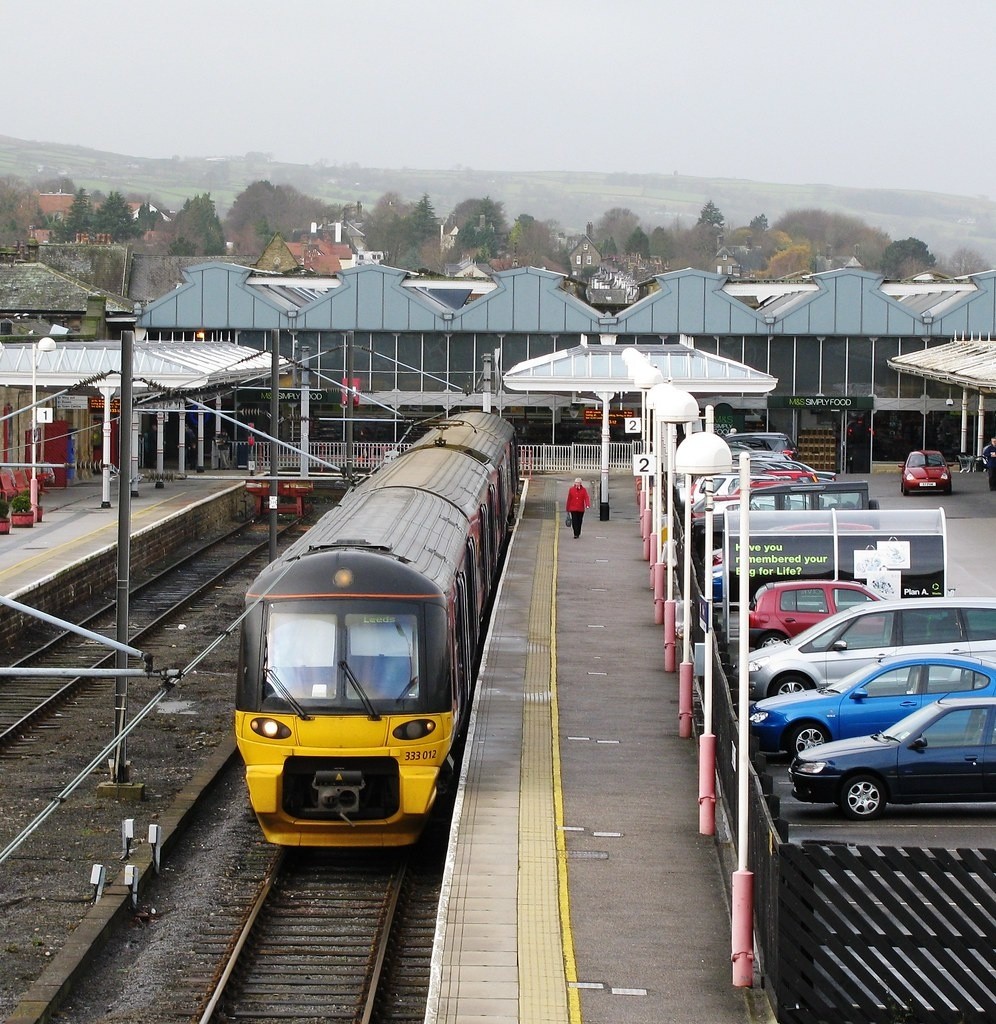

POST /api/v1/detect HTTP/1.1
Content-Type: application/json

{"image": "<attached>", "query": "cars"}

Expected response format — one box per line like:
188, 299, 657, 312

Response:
748, 653, 996, 763
728, 594, 996, 709
748, 575, 935, 651
897, 449, 954, 497
784, 697, 996, 821
666, 431, 880, 603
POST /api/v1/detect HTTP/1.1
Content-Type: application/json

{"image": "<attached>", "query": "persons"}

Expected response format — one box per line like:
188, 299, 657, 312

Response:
982, 438, 996, 491
215, 429, 232, 470
147, 423, 157, 467
566, 478, 590, 539
186, 424, 197, 470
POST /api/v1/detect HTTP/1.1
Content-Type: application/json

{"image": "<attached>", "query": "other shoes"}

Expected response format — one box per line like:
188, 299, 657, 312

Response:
574, 535, 579, 539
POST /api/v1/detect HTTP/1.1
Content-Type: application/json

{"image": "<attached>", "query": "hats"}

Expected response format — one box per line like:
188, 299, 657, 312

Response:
575, 478, 582, 483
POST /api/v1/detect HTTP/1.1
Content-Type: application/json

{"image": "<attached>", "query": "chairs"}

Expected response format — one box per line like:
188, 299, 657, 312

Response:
785, 495, 791, 510
0, 470, 50, 503
963, 714, 985, 745
957, 454, 978, 474
881, 614, 891, 645
904, 666, 921, 694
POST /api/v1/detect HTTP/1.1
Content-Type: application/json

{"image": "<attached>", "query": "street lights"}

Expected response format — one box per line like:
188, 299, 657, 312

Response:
29, 336, 58, 523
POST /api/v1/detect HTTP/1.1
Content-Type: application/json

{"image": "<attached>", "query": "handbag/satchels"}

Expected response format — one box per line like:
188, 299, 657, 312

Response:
565, 512, 571, 527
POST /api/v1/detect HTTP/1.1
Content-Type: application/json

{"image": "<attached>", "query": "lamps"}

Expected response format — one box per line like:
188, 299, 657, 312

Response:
443, 312, 454, 320
288, 309, 298, 318
598, 317, 619, 326
765, 313, 775, 324
922, 315, 934, 324
133, 307, 143, 316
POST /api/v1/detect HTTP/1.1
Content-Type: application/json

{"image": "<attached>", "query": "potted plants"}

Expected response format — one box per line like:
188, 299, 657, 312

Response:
0, 499, 10, 535
11, 496, 33, 528
20, 489, 43, 522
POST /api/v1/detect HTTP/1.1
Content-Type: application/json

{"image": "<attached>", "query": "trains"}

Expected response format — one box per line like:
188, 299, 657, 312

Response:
234, 409, 521, 849
